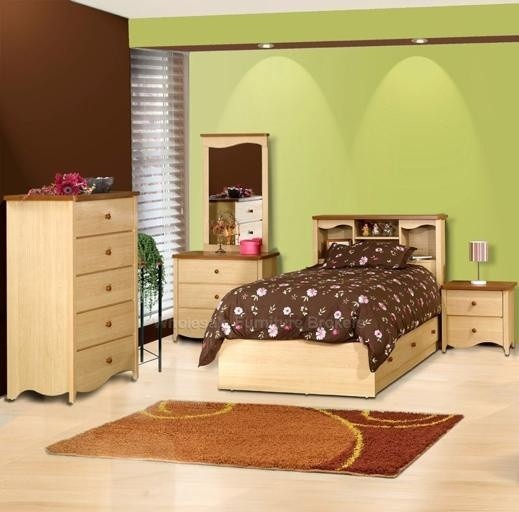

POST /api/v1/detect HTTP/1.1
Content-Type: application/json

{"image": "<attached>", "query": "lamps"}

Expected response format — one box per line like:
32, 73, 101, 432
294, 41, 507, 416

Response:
469, 241, 487, 285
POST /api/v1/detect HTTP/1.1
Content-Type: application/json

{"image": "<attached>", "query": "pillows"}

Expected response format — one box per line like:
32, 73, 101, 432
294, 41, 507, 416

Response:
320, 242, 418, 270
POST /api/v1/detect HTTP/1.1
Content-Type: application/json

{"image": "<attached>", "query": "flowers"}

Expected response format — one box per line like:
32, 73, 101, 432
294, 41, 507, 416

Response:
23, 172, 88, 196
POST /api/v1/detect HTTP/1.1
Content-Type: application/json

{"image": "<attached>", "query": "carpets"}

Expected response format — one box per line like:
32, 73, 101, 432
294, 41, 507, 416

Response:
43, 400, 465, 478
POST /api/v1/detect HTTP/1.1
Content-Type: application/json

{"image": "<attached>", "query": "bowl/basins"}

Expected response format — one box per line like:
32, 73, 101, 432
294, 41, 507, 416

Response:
87, 178, 114, 194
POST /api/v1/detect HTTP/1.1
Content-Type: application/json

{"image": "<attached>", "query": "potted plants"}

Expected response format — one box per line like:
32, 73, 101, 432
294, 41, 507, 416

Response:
137, 231, 167, 319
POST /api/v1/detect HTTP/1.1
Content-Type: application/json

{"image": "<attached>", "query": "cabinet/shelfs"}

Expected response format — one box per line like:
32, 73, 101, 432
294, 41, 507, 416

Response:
209, 195, 263, 246
172, 251, 281, 342
3, 192, 139, 404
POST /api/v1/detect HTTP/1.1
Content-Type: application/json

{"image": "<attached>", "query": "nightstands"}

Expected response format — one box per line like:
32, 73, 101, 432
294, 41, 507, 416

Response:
439, 280, 518, 358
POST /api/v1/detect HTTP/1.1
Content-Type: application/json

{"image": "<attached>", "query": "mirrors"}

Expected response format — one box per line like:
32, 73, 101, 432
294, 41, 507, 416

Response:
200, 132, 270, 253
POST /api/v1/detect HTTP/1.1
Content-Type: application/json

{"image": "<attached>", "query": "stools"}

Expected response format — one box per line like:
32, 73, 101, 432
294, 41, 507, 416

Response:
138, 261, 161, 372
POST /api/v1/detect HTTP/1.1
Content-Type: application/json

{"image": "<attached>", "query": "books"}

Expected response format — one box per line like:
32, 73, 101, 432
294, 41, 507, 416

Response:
412, 255, 433, 260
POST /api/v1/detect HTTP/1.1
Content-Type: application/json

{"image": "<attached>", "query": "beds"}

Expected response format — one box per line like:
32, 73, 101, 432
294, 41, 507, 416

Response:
198, 213, 448, 399
